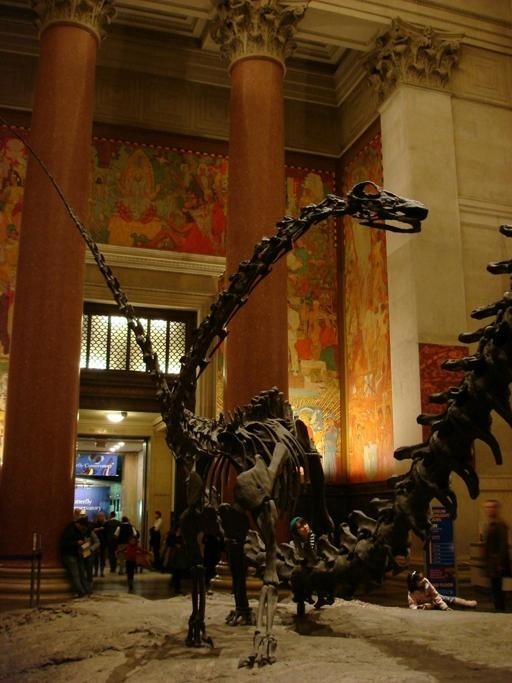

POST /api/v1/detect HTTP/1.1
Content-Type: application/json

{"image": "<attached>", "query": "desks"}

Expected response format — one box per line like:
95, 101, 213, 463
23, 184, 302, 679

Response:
0, 528, 44, 607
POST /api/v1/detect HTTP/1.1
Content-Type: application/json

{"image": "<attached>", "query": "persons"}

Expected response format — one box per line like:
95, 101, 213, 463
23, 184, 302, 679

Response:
290, 516, 339, 623
91, 139, 227, 255
284, 179, 338, 371
58, 506, 222, 598
406, 568, 478, 612
0, 158, 28, 355
478, 498, 509, 611
